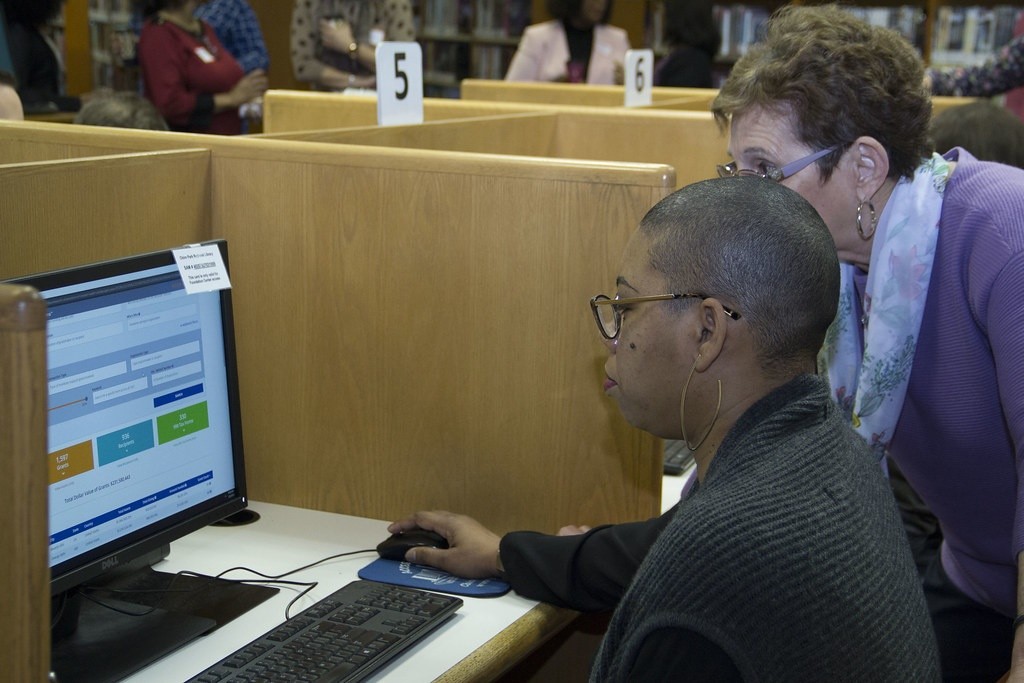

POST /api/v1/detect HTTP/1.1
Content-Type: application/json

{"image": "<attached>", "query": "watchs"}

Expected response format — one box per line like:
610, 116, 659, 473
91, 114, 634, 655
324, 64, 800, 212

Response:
348, 40, 360, 59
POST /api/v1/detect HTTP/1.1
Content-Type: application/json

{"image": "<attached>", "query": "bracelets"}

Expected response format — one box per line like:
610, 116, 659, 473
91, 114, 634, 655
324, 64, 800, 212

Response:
348, 72, 357, 86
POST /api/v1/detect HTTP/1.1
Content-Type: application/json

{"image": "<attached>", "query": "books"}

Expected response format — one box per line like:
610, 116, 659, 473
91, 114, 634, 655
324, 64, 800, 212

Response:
88, 0, 133, 88
715, 5, 768, 57
845, 5, 1017, 52
422, 0, 503, 100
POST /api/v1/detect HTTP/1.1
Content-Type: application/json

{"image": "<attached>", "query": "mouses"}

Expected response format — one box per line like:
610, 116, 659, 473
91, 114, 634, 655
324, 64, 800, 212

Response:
377, 528, 450, 562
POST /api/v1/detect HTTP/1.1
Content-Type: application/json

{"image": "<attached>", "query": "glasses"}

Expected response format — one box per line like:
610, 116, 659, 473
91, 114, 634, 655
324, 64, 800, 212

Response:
716, 141, 850, 182
590, 293, 741, 340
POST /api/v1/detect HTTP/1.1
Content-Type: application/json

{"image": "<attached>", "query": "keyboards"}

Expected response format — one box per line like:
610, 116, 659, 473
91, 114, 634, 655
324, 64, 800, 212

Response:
179, 579, 464, 683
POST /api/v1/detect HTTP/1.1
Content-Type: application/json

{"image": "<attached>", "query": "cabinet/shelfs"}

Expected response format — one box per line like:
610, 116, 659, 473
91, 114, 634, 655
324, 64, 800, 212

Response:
410, 0, 1014, 98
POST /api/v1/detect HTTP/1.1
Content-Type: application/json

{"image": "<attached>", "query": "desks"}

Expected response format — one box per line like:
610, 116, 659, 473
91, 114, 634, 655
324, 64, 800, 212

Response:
117, 497, 580, 683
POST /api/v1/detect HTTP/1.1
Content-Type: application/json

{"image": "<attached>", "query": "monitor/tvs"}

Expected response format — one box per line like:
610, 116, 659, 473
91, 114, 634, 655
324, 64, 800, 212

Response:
0, 240, 279, 683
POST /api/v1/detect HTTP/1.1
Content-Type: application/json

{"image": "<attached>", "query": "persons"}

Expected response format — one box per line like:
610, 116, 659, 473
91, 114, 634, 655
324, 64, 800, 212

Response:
387, 176, 941, 683
709, 0, 1024, 682
505, 0, 631, 86
110, 0, 270, 135
72, 89, 171, 132
290, 0, 418, 92
0, 0, 99, 116
